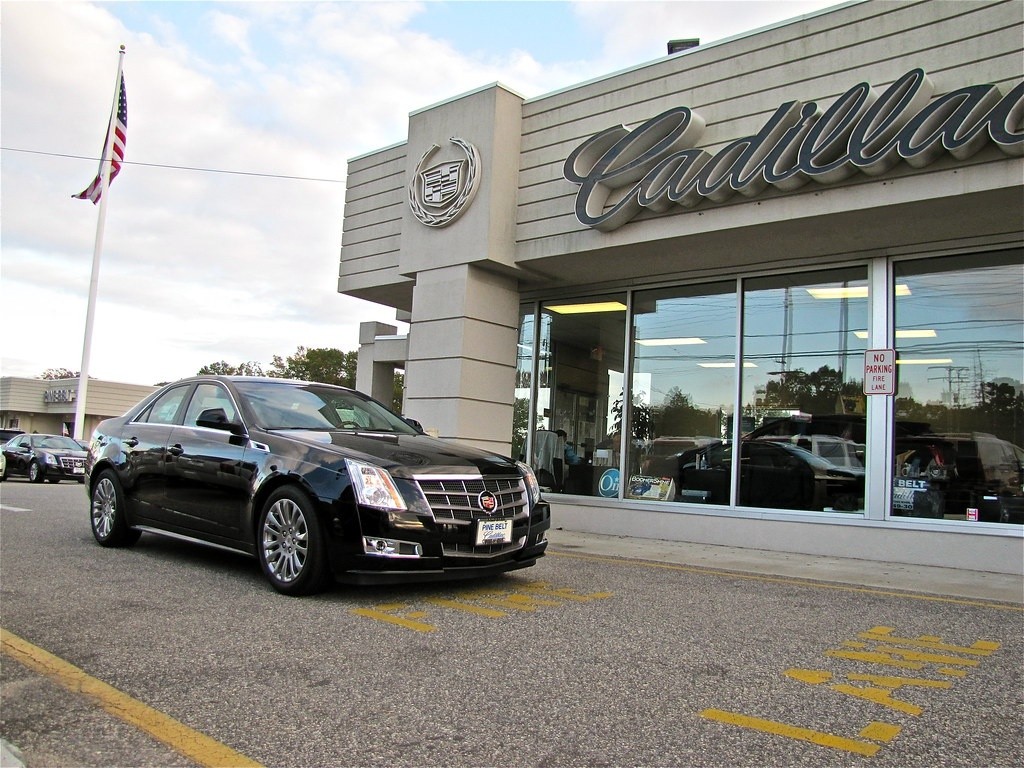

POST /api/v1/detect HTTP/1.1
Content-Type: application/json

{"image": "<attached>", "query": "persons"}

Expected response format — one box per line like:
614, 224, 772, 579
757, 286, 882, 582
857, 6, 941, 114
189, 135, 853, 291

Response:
556, 430, 582, 467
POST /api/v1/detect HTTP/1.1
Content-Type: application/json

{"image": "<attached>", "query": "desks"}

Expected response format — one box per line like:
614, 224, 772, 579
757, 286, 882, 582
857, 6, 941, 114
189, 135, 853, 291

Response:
567, 463, 621, 498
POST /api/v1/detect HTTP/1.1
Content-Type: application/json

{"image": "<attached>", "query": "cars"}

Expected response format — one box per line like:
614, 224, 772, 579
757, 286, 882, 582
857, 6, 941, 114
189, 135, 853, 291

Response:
639, 411, 1024, 524
0, 427, 26, 446
80, 375, 552, 594
0, 433, 88, 485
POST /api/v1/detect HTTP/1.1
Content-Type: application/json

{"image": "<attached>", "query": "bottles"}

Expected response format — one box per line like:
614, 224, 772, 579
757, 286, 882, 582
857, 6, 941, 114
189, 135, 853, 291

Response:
696, 454, 699, 469
700, 454, 706, 470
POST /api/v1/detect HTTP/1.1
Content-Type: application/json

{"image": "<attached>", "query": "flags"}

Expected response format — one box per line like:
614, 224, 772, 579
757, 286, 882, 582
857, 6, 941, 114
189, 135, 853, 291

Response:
72, 71, 128, 205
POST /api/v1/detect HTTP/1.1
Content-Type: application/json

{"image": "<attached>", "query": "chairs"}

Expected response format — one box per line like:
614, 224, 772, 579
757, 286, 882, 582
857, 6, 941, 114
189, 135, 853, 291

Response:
519, 431, 570, 495
